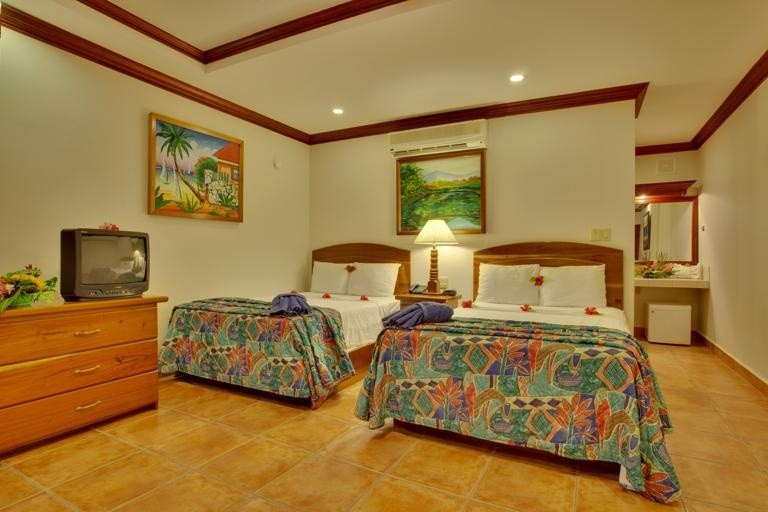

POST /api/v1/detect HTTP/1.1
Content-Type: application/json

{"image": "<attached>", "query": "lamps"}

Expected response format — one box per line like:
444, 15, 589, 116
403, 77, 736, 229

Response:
412, 218, 460, 296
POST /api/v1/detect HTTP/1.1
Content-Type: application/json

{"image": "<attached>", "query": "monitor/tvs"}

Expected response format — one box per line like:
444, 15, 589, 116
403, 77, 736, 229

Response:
60, 228, 150, 302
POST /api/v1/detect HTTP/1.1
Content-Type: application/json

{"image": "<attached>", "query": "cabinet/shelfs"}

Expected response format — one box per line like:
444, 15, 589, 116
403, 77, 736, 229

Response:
0, 292, 169, 456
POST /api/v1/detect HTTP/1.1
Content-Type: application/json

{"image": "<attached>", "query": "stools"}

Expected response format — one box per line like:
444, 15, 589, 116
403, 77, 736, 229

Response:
642, 298, 692, 345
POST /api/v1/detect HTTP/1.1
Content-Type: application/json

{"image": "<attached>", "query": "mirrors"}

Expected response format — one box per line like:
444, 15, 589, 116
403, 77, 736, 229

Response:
633, 194, 700, 267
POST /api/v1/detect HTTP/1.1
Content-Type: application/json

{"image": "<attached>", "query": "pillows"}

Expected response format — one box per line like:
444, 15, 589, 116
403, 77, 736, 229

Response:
309, 258, 353, 296
540, 261, 609, 310
345, 259, 402, 298
472, 261, 540, 308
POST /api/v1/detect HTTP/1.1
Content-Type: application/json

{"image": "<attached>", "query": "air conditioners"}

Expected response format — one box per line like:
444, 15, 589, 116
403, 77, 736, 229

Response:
387, 117, 491, 161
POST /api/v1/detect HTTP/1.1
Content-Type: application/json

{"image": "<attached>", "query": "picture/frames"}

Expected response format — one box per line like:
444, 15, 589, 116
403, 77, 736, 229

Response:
146, 111, 245, 223
395, 147, 489, 235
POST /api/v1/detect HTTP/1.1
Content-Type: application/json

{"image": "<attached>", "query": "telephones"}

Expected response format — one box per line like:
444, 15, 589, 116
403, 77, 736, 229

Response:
409, 283, 427, 294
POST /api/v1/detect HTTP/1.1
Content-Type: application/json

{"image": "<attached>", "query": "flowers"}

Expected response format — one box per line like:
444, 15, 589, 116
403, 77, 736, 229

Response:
0, 263, 58, 313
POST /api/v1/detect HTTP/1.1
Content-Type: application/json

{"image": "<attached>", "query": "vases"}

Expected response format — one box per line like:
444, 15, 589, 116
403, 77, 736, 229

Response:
8, 293, 33, 307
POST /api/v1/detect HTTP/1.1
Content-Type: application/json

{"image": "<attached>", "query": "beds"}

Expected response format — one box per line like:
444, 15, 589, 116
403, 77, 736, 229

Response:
391, 240, 640, 473
174, 241, 412, 405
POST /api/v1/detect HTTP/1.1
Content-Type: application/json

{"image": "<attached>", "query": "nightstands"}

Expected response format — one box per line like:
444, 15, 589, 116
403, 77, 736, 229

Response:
393, 292, 463, 311
